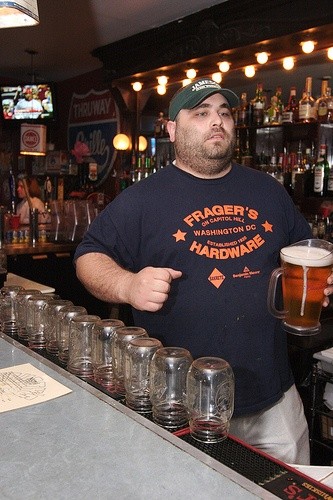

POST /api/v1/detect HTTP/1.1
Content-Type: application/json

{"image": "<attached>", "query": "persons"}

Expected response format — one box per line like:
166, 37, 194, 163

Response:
74, 78, 333, 467
17, 176, 44, 225
2, 85, 54, 120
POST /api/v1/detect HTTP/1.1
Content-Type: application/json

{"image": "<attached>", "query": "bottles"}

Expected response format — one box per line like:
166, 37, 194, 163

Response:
91, 318, 125, 387
15, 290, 43, 339
0, 286, 25, 333
111, 326, 148, 396
0, 109, 176, 273
55, 305, 88, 363
183, 356, 235, 444
43, 299, 74, 356
148, 345, 192, 429
26, 295, 55, 348
232, 77, 333, 242
123, 337, 162, 413
67, 314, 101, 378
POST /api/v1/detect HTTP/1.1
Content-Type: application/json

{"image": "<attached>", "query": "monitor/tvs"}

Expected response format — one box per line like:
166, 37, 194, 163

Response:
0, 81, 57, 124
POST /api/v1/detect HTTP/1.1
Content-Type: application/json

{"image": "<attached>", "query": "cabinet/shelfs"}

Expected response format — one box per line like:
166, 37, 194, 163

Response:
89, 0, 333, 243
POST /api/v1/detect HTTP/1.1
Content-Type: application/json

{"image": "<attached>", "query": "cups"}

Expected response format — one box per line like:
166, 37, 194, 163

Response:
267, 237, 333, 338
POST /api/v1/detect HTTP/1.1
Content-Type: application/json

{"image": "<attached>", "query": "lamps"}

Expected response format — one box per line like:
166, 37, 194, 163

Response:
112, 134, 130, 161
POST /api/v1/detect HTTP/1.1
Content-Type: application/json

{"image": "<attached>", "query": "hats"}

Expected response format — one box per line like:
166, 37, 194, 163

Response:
168, 78, 241, 121
